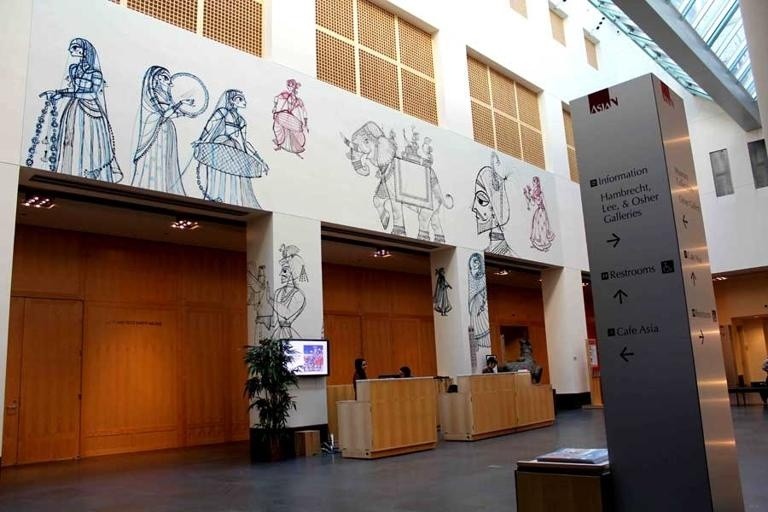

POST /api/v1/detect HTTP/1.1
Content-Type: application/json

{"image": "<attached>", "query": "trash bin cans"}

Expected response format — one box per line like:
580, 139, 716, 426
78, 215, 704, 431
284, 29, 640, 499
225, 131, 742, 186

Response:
512, 457, 615, 512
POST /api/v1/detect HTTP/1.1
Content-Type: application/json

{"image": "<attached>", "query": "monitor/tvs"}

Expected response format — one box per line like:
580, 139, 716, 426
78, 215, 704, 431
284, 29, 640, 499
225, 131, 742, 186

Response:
280, 339, 330, 378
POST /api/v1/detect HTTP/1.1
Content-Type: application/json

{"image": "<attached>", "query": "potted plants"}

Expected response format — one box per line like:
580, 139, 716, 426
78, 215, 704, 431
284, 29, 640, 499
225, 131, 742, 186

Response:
242, 336, 299, 463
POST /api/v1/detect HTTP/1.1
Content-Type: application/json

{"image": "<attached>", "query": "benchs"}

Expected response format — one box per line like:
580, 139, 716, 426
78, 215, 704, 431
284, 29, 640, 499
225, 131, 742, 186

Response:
728, 387, 768, 407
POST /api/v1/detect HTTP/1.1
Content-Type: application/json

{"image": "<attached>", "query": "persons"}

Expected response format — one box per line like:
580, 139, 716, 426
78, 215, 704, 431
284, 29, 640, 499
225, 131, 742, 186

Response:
353, 359, 368, 400
399, 367, 411, 378
483, 357, 497, 373
